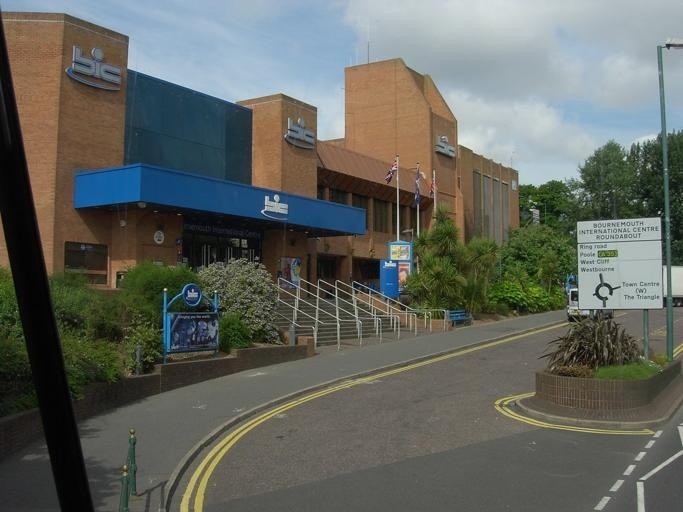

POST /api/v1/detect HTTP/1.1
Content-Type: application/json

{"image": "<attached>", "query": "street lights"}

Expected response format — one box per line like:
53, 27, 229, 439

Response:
653, 36, 681, 373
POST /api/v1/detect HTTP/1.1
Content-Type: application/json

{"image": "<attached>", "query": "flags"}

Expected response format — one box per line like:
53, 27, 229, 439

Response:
412, 168, 423, 208
428, 175, 436, 197
387, 160, 397, 184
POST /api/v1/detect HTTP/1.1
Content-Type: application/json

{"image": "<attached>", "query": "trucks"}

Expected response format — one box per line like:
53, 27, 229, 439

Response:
653, 260, 683, 307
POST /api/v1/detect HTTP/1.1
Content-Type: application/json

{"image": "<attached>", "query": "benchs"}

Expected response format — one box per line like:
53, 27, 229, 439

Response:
450, 310, 473, 327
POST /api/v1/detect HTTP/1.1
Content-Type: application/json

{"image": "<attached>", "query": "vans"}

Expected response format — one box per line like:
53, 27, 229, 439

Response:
565, 286, 616, 322
